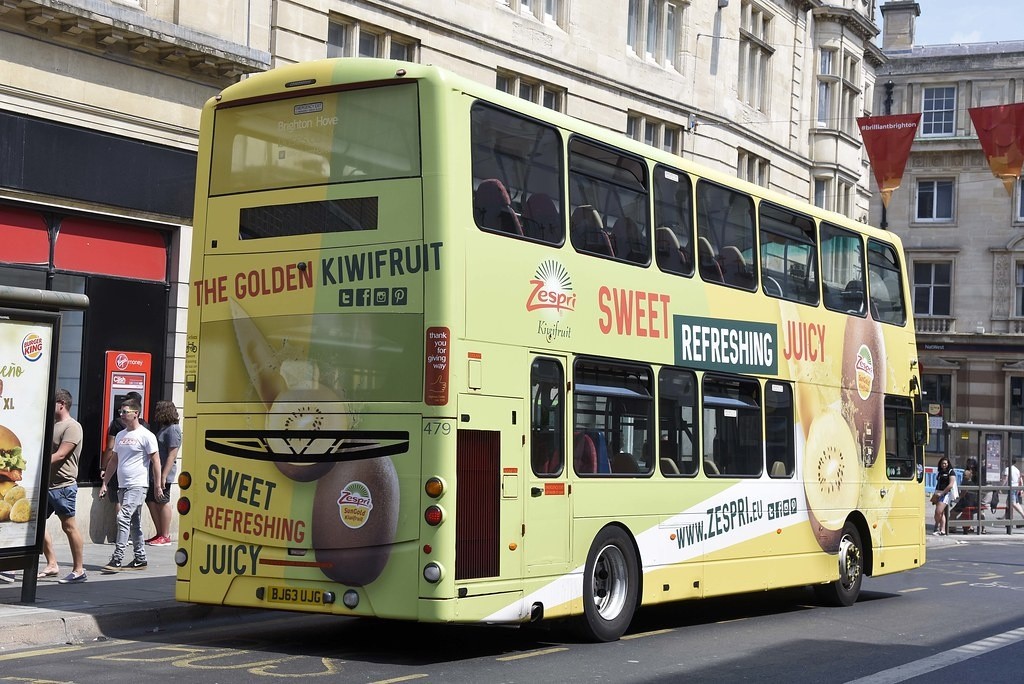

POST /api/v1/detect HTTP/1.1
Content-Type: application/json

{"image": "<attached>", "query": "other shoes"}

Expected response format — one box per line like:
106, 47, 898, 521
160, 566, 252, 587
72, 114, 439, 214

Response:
38, 572, 59, 578
932, 523, 946, 536
59, 569, 87, 584
124, 537, 134, 546
981, 529, 986, 533
968, 527, 974, 533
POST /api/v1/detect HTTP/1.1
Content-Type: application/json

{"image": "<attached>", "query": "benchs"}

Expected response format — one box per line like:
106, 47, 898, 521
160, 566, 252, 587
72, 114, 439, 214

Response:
950, 506, 1008, 533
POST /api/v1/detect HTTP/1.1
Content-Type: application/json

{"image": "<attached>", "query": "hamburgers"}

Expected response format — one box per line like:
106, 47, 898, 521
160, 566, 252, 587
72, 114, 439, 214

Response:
0, 425, 27, 500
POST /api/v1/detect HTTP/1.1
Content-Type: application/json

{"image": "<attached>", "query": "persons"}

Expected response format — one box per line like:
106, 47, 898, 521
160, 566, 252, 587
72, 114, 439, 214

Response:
100, 392, 150, 545
930, 454, 1022, 535
37, 389, 87, 585
99, 398, 164, 572
145, 400, 181, 547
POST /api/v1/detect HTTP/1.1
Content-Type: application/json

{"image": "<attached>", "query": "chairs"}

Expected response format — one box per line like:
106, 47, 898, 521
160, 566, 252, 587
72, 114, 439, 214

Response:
533, 428, 787, 477
474, 178, 883, 322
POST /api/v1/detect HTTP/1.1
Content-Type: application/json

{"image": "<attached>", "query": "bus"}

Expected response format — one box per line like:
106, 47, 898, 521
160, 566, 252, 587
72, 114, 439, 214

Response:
175, 56, 929, 644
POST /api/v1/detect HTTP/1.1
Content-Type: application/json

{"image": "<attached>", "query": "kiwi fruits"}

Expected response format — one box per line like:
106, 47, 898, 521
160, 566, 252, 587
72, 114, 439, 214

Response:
841, 313, 887, 467
264, 381, 348, 482
803, 409, 861, 555
311, 439, 400, 588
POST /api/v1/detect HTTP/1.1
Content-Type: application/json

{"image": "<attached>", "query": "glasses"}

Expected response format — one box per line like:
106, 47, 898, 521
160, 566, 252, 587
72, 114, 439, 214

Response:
118, 410, 138, 414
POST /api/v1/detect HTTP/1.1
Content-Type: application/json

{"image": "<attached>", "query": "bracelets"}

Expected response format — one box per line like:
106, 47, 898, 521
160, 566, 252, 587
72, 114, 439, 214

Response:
100, 467, 106, 471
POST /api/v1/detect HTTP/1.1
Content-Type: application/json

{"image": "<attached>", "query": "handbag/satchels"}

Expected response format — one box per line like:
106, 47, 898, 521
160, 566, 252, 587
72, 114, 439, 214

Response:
1019, 497, 1022, 504
930, 494, 939, 504
948, 469, 959, 500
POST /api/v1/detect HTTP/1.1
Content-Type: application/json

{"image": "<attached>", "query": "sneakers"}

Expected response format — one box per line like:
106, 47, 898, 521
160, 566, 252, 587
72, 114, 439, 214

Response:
150, 535, 171, 546
121, 559, 147, 570
145, 535, 161, 544
101, 556, 121, 572
